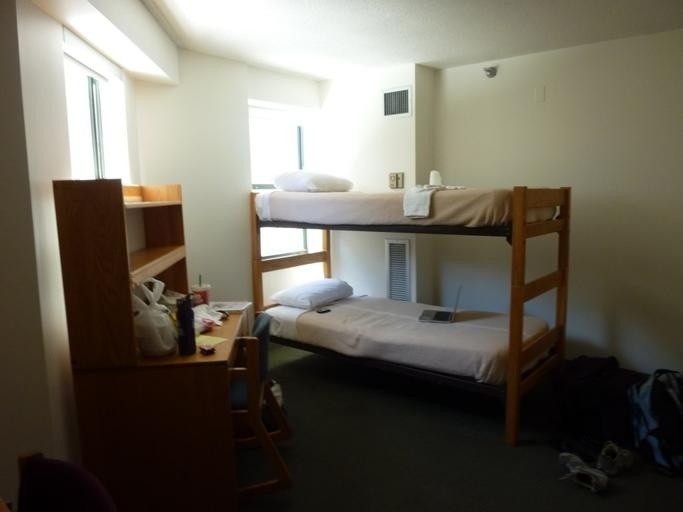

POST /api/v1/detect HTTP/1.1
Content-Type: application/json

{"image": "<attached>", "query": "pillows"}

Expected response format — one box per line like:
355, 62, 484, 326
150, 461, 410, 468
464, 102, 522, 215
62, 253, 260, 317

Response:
269, 278, 353, 310
273, 171, 353, 192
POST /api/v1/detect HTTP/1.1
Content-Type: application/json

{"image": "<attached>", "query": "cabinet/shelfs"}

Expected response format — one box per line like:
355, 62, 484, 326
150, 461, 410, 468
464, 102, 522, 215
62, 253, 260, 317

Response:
51, 178, 290, 512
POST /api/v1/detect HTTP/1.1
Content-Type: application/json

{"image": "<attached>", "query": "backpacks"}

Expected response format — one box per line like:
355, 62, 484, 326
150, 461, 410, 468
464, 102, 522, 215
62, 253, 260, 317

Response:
628, 369, 682, 475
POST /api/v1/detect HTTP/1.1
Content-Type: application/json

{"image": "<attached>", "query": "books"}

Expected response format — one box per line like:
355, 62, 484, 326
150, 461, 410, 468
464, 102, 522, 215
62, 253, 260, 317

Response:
209, 301, 252, 314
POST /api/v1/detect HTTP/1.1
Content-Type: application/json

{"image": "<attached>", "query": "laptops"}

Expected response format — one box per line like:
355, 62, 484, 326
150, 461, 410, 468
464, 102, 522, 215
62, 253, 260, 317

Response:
416, 285, 462, 324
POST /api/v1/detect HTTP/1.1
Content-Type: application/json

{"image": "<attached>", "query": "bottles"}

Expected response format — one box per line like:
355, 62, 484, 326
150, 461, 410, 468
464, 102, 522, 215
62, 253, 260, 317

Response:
176, 299, 197, 355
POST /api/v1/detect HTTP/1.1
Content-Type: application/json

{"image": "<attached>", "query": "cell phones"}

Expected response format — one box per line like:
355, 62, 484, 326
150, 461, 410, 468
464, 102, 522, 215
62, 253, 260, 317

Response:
316, 307, 331, 313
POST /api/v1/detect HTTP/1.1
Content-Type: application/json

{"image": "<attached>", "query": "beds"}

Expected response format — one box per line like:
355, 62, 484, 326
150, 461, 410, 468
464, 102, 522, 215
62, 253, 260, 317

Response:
248, 184, 571, 445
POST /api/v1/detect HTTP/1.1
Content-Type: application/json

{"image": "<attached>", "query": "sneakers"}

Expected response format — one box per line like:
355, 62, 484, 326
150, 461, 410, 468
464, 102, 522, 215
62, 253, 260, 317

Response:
597, 441, 632, 477
559, 453, 609, 492
